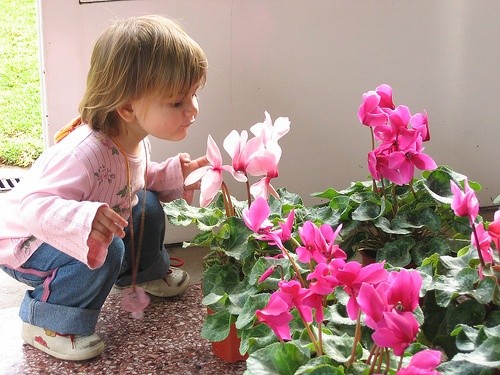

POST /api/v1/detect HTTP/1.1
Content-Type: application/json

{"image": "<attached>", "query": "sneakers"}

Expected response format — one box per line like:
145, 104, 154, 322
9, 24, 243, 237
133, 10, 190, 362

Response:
21, 321, 105, 361
112, 265, 191, 298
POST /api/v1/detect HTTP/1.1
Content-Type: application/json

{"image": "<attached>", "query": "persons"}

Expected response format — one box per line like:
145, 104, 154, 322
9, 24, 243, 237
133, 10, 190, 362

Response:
0, 30, 211, 361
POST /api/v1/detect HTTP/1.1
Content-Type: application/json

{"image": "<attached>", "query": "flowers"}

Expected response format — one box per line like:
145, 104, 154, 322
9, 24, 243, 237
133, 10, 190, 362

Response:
160, 83, 500, 375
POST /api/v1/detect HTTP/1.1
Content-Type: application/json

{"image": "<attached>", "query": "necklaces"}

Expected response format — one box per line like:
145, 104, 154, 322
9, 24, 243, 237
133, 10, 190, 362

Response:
103, 122, 152, 320
